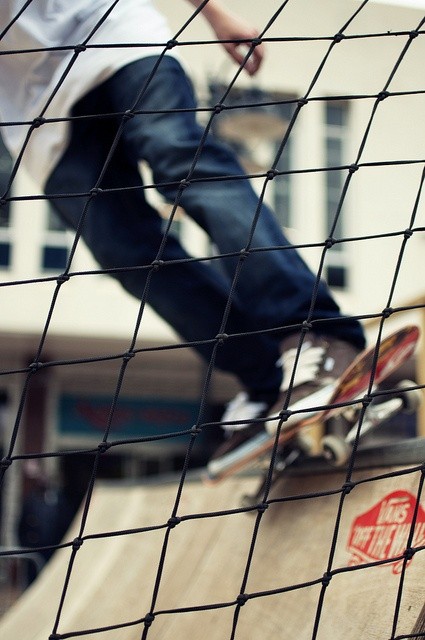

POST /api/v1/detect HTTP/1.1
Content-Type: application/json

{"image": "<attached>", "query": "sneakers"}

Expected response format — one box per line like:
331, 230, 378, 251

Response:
207, 392, 298, 479
264, 332, 357, 434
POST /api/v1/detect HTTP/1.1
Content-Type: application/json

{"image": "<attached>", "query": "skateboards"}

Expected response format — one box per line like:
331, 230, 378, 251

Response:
207, 325, 421, 534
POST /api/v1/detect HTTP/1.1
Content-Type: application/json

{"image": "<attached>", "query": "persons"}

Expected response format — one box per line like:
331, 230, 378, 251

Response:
0, 1, 364, 437
